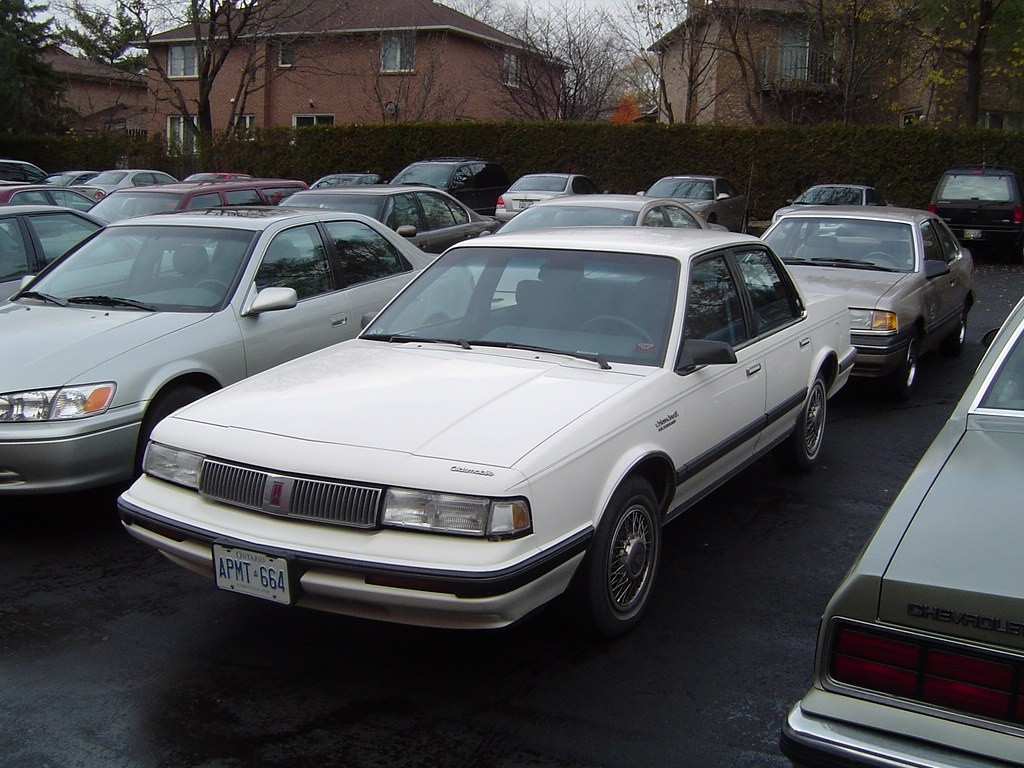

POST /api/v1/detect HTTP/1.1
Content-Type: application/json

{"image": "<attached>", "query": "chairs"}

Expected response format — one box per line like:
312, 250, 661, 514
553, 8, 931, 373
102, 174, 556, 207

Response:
795, 236, 910, 268
158, 245, 210, 294
512, 279, 676, 342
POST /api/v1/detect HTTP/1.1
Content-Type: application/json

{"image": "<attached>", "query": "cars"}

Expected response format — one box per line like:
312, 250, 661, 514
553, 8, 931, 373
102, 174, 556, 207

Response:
779, 294, 1024, 768
183, 172, 253, 182
0, 159, 48, 187
621, 174, 747, 235
925, 166, 1024, 261
771, 184, 893, 239
493, 174, 613, 224
0, 210, 477, 496
1, 204, 215, 311
115, 225, 859, 640
0, 185, 98, 213
308, 173, 390, 189
465, 192, 731, 310
30, 171, 101, 187
735, 206, 978, 402
76, 170, 179, 204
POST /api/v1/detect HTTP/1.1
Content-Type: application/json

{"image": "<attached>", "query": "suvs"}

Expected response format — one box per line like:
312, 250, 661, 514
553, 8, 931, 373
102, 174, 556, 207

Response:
389, 155, 511, 218
88, 178, 307, 225
